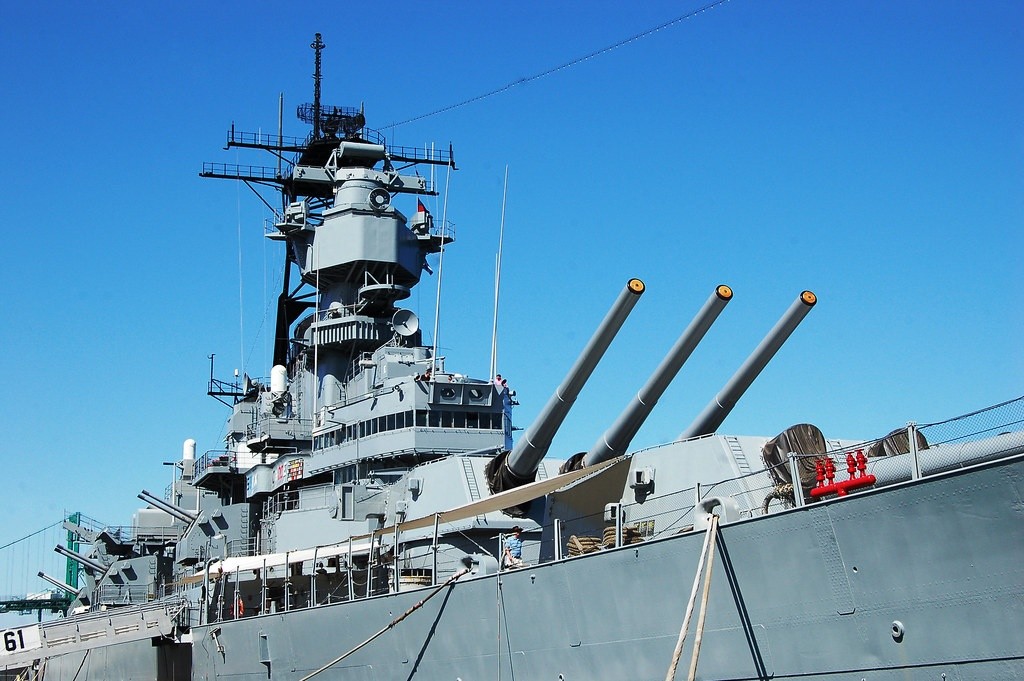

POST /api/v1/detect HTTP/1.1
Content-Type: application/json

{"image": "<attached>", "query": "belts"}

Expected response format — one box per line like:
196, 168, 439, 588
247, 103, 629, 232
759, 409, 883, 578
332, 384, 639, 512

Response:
512, 556, 520, 559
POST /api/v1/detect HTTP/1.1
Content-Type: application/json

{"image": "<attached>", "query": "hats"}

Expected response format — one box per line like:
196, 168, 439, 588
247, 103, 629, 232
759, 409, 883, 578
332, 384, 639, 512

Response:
511, 526, 523, 535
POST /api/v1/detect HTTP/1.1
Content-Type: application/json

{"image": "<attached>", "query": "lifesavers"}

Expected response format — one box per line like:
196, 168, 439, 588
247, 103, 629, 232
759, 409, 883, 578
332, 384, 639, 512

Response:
230, 598, 244, 616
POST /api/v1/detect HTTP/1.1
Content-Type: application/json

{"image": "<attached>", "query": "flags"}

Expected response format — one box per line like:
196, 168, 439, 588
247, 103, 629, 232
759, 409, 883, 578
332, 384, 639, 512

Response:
418, 198, 430, 213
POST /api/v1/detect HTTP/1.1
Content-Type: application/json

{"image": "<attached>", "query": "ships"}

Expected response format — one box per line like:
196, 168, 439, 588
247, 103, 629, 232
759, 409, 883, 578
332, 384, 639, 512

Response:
0, 33, 1024, 681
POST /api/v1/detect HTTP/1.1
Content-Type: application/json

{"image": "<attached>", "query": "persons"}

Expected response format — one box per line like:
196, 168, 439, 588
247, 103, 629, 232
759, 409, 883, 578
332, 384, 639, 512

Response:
421, 370, 430, 381
504, 526, 523, 569
496, 374, 508, 387
